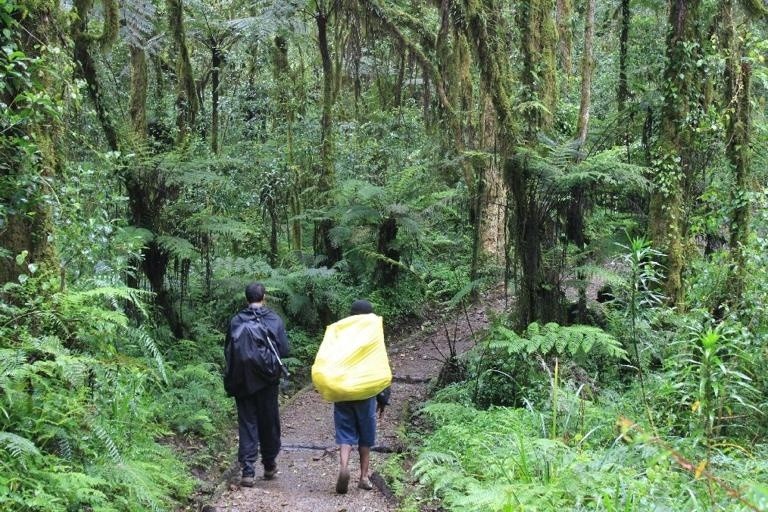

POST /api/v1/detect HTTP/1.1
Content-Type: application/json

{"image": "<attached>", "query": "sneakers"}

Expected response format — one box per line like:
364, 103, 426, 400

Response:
240, 472, 255, 486
358, 480, 373, 490
336, 469, 350, 494
264, 464, 279, 479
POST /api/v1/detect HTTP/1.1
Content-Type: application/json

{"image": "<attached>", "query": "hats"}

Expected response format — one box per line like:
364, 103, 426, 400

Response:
350, 301, 373, 315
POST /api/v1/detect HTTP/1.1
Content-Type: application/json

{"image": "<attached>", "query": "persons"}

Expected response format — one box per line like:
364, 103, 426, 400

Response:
224, 283, 292, 488
334, 300, 391, 495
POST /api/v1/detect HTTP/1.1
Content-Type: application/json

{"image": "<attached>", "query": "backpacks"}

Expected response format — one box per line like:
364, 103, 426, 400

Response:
224, 316, 283, 400
